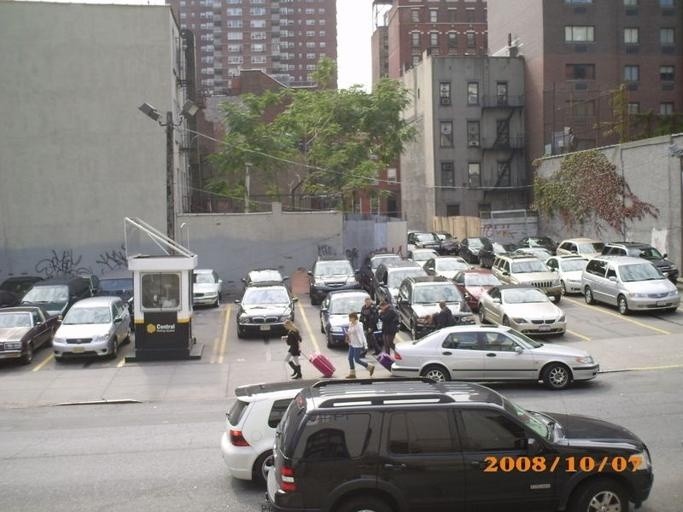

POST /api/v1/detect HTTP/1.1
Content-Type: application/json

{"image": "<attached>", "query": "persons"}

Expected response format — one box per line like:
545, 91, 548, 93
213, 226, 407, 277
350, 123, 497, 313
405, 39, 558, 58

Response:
284, 319, 302, 380
378, 302, 401, 355
437, 300, 452, 327
359, 298, 382, 356
344, 312, 375, 378
421, 314, 436, 335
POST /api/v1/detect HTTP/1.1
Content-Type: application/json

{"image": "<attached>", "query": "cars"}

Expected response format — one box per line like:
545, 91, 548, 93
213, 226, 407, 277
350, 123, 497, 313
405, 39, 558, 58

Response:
234, 281, 299, 339
476, 284, 566, 336
541, 254, 590, 297
319, 289, 384, 348
216, 375, 317, 486
386, 322, 602, 391
240, 268, 290, 292
450, 268, 503, 311
192, 268, 224, 309
406, 229, 559, 279
0, 268, 133, 368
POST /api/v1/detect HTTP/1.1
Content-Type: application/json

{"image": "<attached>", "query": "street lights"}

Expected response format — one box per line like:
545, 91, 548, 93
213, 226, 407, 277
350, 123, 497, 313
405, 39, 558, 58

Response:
138, 100, 176, 258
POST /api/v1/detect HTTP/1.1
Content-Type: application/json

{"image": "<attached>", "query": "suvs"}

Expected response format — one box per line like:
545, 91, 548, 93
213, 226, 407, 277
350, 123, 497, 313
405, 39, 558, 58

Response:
369, 260, 430, 306
395, 277, 476, 340
360, 252, 403, 294
306, 255, 361, 305
555, 237, 605, 257
490, 252, 563, 303
600, 241, 679, 286
580, 255, 681, 316
258, 376, 655, 512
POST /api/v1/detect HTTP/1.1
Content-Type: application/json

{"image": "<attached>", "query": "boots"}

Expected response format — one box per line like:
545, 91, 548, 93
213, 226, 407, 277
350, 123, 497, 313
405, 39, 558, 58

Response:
365, 363, 374, 375
346, 368, 356, 378
289, 361, 302, 378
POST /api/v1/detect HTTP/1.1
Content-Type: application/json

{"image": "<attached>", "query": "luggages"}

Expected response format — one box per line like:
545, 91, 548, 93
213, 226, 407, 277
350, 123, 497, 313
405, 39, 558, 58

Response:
299, 350, 335, 377
366, 350, 395, 371
